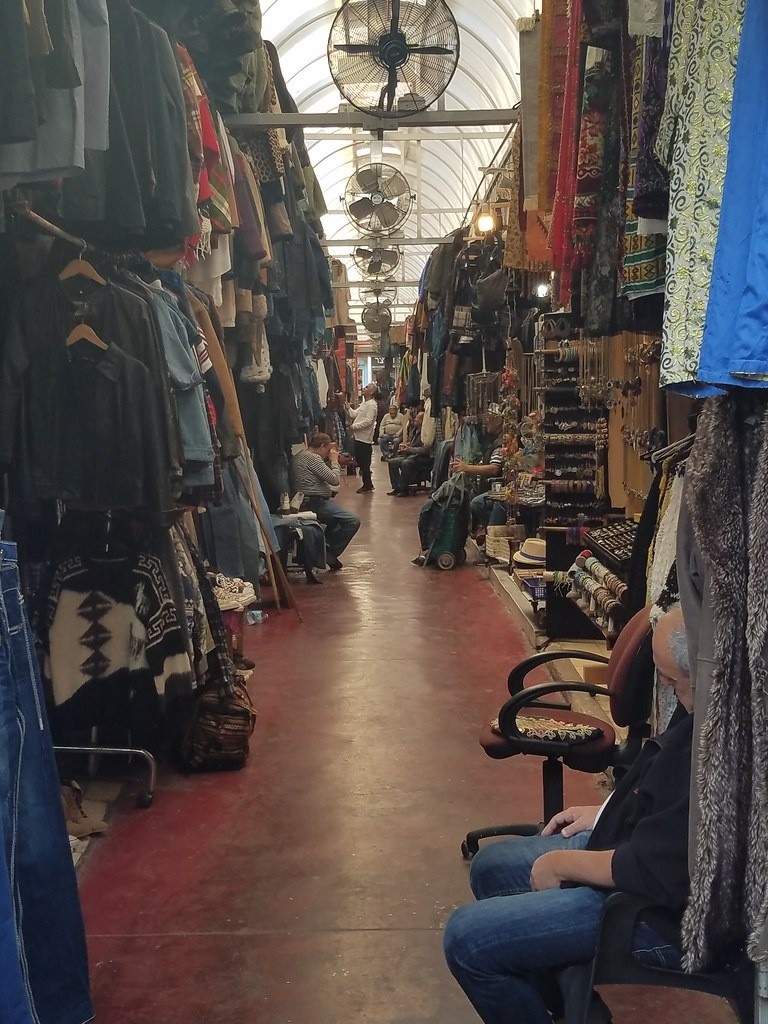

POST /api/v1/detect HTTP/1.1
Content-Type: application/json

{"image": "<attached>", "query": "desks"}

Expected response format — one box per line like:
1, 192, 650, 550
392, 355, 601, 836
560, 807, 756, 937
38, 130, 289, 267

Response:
484, 486, 545, 537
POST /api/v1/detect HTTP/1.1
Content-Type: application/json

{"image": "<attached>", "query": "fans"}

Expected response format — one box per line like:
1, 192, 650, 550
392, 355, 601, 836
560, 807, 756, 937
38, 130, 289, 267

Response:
357, 274, 397, 310
350, 233, 404, 280
339, 162, 416, 237
360, 303, 392, 333
327, 0, 461, 118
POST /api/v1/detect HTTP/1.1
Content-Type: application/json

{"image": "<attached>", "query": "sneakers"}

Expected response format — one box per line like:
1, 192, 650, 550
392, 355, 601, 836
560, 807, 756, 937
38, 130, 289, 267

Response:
207, 572, 256, 612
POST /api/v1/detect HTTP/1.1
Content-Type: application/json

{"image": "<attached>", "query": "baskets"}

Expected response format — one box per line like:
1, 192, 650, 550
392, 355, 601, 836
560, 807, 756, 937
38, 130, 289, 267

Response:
523, 579, 546, 599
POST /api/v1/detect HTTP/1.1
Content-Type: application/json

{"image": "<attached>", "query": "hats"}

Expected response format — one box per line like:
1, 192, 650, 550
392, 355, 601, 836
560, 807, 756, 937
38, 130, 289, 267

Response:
488, 403, 504, 417
513, 538, 546, 565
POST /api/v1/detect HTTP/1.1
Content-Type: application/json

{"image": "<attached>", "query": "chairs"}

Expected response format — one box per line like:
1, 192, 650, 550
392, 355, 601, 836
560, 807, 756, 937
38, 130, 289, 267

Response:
462, 604, 652, 857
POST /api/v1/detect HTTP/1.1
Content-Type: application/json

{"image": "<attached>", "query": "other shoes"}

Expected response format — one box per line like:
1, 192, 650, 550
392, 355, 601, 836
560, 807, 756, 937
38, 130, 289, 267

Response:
473, 554, 499, 566
64, 785, 108, 833
59, 796, 92, 839
372, 485, 375, 490
357, 485, 372, 493
325, 552, 343, 569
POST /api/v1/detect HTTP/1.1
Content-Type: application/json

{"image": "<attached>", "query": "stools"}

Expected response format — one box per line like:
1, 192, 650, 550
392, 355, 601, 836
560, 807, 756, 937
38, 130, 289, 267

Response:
408, 472, 431, 495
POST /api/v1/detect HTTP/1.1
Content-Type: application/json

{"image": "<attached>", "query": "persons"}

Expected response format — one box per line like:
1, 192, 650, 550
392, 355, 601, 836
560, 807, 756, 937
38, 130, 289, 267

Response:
377, 405, 403, 461
343, 383, 380, 495
386, 412, 434, 497
292, 433, 360, 570
449, 418, 505, 565
443, 602, 693, 1024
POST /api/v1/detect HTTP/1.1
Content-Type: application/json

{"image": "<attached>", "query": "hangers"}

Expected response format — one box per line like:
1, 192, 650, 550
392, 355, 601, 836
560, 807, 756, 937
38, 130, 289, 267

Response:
66, 315, 109, 351
639, 434, 696, 478
59, 239, 108, 286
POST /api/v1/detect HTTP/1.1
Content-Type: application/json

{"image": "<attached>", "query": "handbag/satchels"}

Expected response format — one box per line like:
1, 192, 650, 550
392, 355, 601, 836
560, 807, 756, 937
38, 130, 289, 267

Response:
172, 675, 255, 771
331, 392, 345, 407
476, 269, 505, 309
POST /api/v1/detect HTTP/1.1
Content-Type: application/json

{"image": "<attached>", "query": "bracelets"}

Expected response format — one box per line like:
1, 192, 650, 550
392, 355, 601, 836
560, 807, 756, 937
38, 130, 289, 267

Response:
551, 479, 594, 494
580, 560, 628, 613
554, 347, 573, 363
